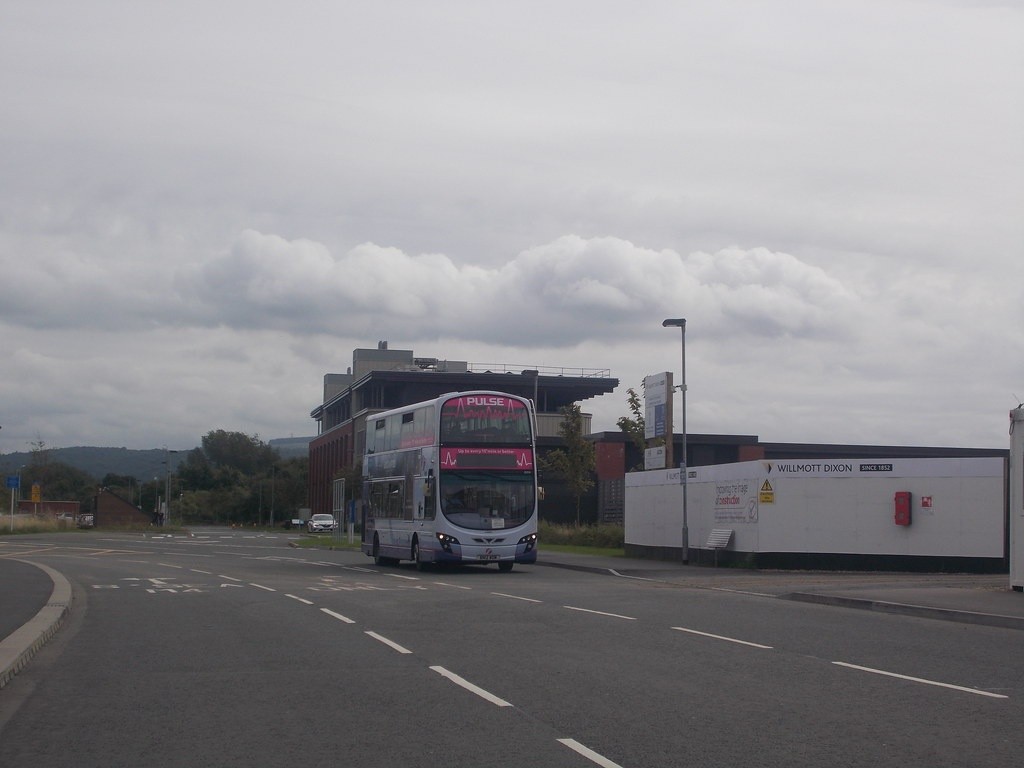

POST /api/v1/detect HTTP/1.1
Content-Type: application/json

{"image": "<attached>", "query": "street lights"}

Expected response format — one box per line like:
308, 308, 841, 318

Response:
14, 465, 27, 513
126, 446, 186, 530
662, 316, 692, 566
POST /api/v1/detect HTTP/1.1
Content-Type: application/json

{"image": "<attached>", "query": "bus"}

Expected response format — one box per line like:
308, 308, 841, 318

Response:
361, 390, 544, 573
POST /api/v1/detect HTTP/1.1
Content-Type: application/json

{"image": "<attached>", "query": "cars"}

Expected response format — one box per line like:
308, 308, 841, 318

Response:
308, 513, 338, 533
